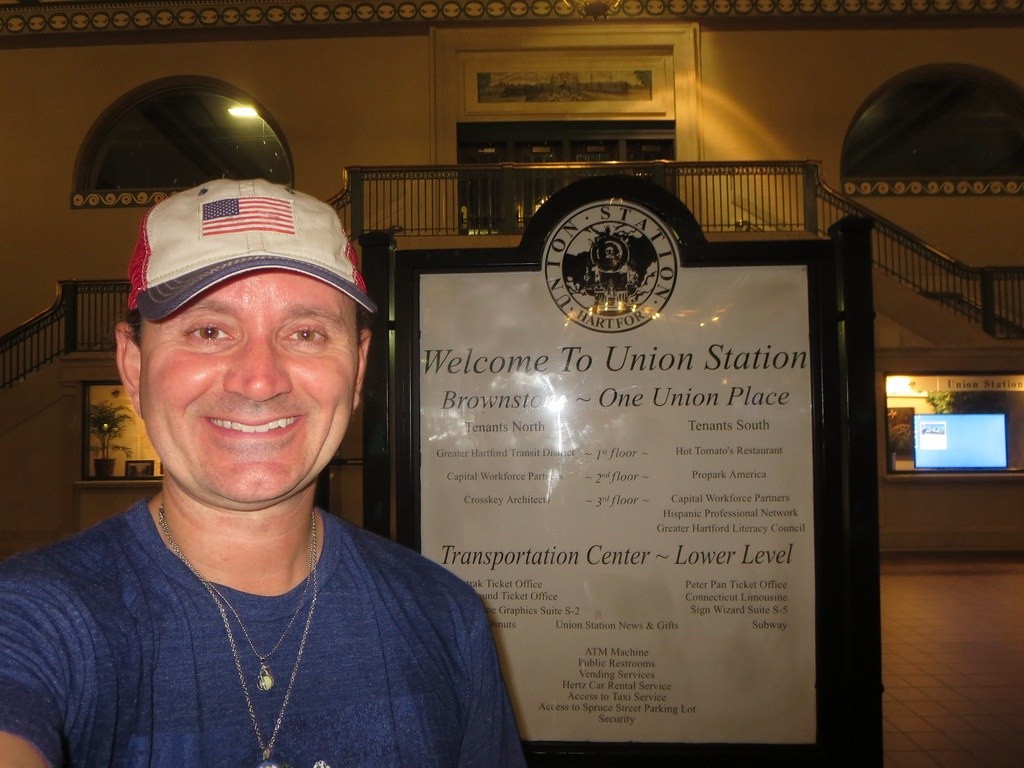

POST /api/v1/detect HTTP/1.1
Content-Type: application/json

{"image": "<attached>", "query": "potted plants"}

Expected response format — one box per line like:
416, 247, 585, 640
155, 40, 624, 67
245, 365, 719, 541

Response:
89, 400, 127, 475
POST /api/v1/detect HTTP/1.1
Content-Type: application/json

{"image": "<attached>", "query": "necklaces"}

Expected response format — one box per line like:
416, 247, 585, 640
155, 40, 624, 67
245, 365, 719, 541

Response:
159, 499, 319, 767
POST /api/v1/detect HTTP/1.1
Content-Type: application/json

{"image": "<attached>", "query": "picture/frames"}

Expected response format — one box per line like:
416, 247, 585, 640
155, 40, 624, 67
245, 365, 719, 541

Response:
457, 46, 670, 117
124, 458, 155, 477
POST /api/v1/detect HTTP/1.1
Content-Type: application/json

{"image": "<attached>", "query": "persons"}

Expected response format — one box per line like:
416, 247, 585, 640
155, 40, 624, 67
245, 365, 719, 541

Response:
0, 178, 530, 767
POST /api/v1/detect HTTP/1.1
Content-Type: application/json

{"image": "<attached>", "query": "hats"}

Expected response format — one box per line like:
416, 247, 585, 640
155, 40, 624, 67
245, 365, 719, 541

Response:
128, 179, 378, 321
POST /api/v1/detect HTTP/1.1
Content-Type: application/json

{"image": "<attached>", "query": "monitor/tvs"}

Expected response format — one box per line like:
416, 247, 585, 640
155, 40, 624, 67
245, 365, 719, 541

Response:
912, 412, 1008, 470
